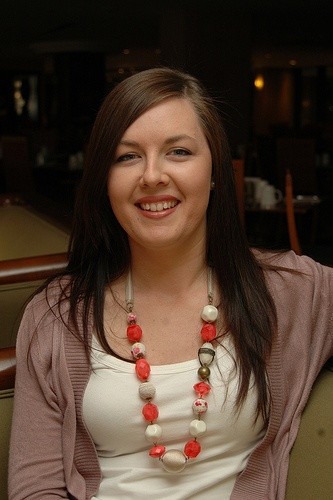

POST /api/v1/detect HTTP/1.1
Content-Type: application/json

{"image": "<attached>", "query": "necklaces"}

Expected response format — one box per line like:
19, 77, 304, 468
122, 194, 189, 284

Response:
125, 265, 219, 473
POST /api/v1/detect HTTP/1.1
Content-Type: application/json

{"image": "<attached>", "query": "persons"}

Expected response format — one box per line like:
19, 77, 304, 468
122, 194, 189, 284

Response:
7, 65, 333, 500
